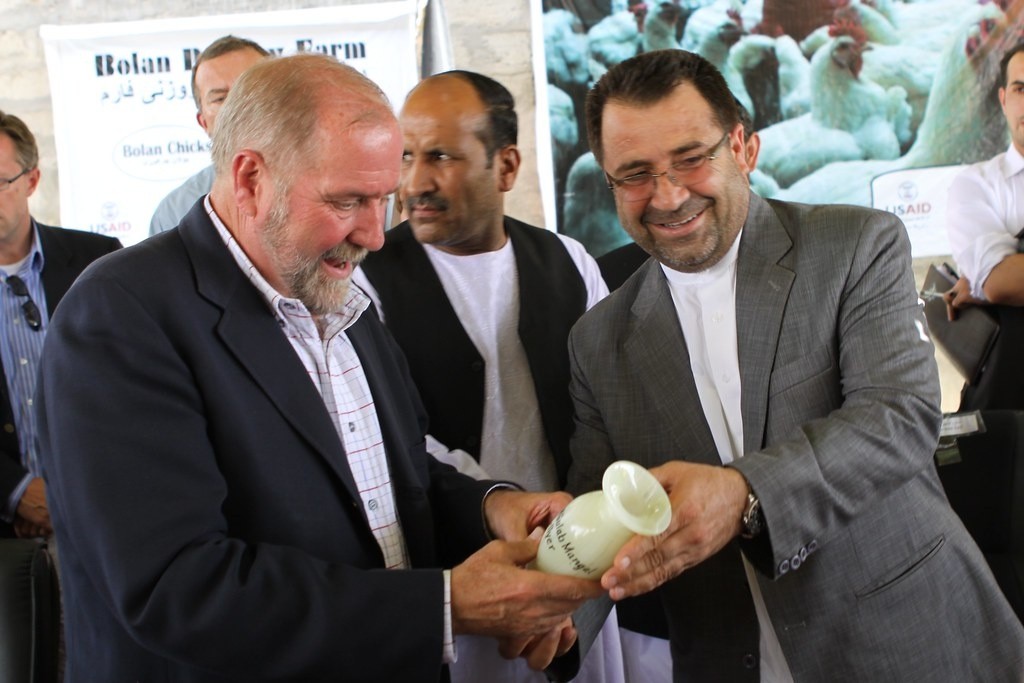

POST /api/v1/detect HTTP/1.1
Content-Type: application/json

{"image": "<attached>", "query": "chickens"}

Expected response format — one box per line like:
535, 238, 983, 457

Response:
540, 0, 1013, 262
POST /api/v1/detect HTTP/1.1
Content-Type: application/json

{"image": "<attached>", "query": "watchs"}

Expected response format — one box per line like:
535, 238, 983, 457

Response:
736, 475, 767, 539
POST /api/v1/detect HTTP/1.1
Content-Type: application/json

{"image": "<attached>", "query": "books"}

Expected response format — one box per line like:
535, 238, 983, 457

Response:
915, 262, 999, 385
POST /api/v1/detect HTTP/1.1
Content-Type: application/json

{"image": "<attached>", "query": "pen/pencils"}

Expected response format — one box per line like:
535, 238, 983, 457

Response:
942, 262, 962, 282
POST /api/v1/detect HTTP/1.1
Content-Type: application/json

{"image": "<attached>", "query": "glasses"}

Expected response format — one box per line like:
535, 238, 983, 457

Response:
604, 127, 730, 202
6, 275, 41, 332
0, 168, 28, 191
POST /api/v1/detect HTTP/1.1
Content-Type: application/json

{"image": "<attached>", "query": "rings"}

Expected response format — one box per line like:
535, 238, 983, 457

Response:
948, 289, 957, 298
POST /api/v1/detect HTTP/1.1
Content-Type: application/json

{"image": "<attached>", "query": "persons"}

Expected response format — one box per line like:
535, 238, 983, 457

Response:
1, 28, 1024, 683
